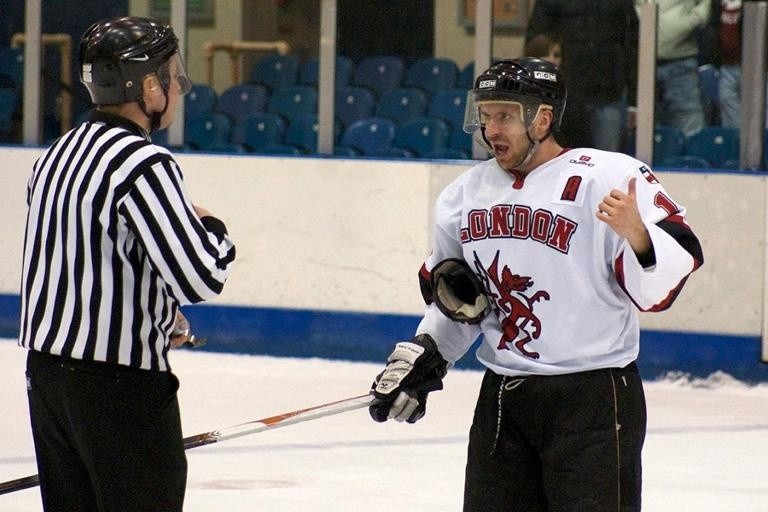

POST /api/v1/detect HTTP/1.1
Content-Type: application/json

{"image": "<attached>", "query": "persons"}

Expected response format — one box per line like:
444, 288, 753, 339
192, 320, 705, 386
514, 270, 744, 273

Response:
369, 55, 705, 511
630, 0, 713, 140
17, 16, 234, 512
707, 0, 743, 132
527, 1, 634, 154
526, 32, 563, 73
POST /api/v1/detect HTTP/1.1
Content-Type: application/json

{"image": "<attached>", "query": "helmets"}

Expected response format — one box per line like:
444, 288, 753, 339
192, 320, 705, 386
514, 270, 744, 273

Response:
461, 53, 568, 137
77, 15, 192, 104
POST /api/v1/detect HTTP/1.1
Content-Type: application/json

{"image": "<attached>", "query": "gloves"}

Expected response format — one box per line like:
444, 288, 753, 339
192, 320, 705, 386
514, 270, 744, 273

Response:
431, 256, 490, 327
370, 333, 449, 425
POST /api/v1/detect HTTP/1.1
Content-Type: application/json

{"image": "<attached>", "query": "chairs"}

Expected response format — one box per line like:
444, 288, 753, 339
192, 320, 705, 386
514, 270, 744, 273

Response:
522, 32, 766, 172
149, 50, 503, 159
0, 0, 131, 151
